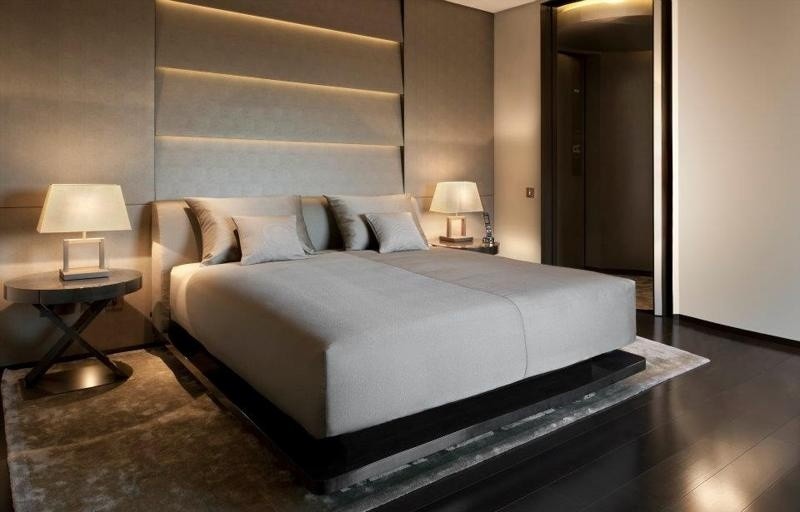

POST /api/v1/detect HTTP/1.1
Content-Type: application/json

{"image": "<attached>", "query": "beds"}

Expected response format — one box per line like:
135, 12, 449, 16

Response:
150, 191, 646, 497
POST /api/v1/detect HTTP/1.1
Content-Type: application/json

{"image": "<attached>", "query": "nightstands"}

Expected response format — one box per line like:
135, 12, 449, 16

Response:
431, 241, 501, 255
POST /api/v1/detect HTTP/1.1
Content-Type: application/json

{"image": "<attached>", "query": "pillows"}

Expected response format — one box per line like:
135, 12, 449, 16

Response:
182, 195, 315, 265
322, 193, 428, 251
363, 211, 430, 254
230, 215, 306, 266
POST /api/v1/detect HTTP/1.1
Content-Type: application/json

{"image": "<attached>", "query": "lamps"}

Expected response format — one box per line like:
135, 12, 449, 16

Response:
37, 183, 133, 281
429, 181, 484, 242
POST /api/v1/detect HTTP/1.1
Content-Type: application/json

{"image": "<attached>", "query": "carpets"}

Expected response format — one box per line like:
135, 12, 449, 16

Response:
0, 335, 710, 512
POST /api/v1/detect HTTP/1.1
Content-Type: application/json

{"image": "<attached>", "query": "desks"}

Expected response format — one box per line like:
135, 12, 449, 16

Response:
4, 268, 143, 401
